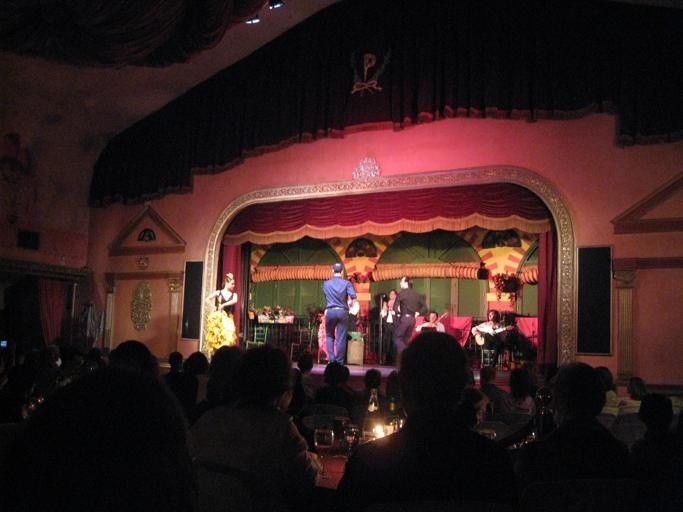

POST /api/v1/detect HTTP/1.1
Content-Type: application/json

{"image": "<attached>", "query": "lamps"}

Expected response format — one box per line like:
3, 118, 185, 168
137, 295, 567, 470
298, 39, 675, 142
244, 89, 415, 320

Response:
246, 15, 261, 24
269, 0, 285, 10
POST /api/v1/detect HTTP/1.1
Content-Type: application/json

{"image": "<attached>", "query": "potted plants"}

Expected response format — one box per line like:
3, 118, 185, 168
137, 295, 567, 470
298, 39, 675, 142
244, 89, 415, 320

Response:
493, 275, 524, 308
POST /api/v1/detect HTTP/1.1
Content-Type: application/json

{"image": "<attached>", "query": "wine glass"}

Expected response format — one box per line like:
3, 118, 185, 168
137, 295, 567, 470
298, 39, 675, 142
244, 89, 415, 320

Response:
334, 417, 345, 458
314, 429, 335, 481
341, 424, 359, 458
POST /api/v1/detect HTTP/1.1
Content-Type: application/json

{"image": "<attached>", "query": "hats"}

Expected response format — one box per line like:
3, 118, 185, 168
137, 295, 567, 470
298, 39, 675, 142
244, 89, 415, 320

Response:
332, 263, 343, 273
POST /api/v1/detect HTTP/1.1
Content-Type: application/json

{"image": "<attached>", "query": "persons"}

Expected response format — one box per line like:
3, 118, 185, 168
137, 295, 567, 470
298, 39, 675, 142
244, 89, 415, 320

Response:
316, 308, 337, 362
378, 288, 400, 366
200, 272, 238, 362
415, 311, 446, 334
321, 263, 357, 368
390, 274, 428, 357
1, 331, 683, 512
469, 309, 514, 369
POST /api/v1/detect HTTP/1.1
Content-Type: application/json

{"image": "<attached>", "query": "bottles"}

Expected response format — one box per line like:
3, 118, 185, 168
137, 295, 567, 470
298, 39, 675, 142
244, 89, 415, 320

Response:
367, 388, 380, 417
384, 397, 397, 434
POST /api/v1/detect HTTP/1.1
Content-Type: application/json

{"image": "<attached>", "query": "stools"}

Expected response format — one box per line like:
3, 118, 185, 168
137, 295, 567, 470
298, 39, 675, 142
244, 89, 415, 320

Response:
481, 348, 503, 369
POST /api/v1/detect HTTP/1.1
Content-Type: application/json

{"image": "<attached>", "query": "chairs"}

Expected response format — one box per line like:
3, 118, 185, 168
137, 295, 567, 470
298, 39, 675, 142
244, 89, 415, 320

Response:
289, 320, 316, 360
245, 325, 269, 351
